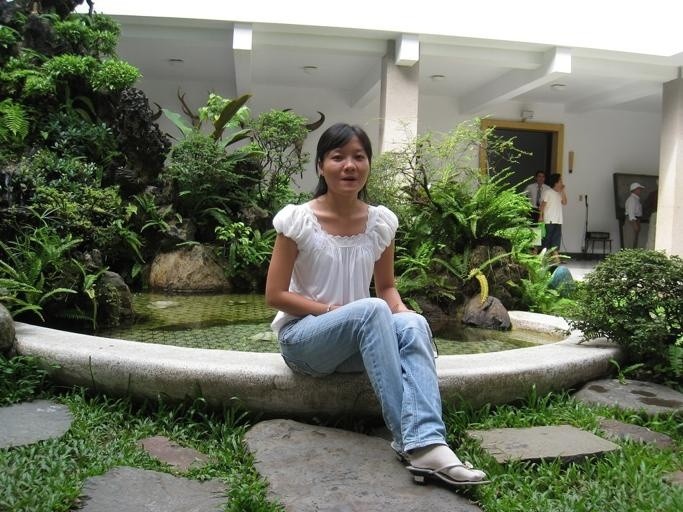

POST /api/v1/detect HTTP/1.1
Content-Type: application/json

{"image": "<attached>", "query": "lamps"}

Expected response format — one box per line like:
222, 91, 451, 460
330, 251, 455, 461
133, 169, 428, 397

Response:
568, 152, 575, 173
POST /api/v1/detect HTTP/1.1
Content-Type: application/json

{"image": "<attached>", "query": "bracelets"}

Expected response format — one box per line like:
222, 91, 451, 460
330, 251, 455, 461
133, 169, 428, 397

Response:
326, 303, 332, 313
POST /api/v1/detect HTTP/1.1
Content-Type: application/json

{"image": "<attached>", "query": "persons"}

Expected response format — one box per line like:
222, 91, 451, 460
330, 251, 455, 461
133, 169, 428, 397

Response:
264, 122, 491, 489
538, 173, 567, 266
521, 172, 549, 226
622, 182, 646, 248
642, 179, 658, 253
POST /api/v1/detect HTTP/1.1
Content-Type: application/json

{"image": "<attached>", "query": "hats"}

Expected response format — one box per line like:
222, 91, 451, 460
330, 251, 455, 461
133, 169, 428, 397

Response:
629, 182, 644, 191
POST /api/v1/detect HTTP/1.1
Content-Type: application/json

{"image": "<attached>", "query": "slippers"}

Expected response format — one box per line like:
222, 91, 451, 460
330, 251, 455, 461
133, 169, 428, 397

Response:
404, 461, 491, 485
390, 441, 408, 464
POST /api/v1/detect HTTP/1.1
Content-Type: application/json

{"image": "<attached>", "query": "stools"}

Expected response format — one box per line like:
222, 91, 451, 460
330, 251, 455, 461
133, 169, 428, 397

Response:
585, 232, 611, 260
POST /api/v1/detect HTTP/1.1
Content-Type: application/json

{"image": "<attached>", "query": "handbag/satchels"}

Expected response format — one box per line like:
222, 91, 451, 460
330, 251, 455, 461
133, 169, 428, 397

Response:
530, 227, 541, 246
